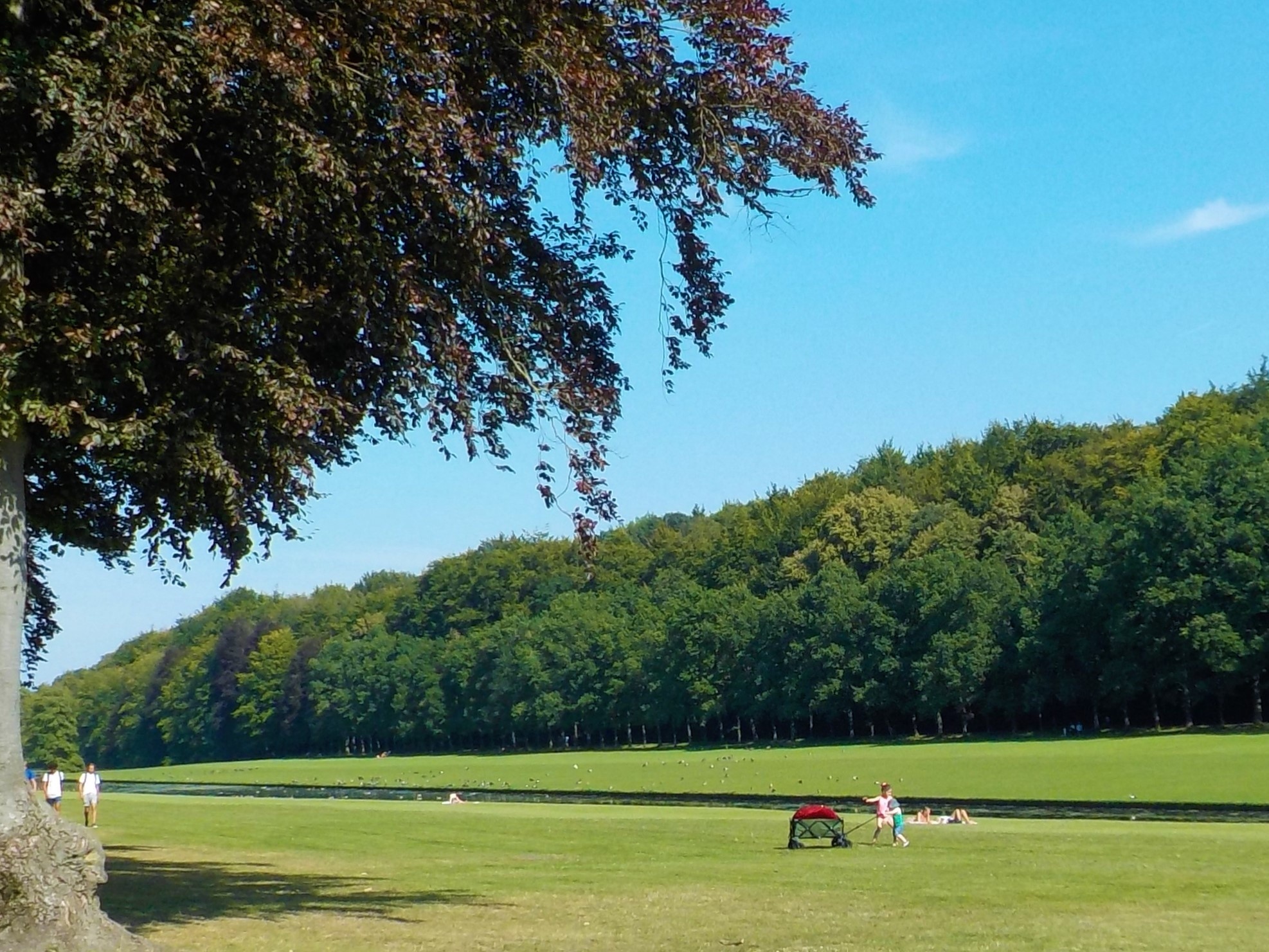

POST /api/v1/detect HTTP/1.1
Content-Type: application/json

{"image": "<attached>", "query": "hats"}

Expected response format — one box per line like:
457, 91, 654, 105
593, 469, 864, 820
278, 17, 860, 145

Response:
887, 798, 900, 808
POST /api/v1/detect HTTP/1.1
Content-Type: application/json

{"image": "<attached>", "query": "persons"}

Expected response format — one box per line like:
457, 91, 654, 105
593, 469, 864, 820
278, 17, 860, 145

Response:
881, 799, 910, 848
42, 761, 64, 812
78, 762, 101, 828
23, 761, 37, 792
905, 806, 931, 823
862, 783, 895, 842
934, 808, 978, 825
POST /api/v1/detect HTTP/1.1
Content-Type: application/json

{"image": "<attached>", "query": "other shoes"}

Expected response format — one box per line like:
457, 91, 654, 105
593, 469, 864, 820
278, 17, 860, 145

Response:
903, 841, 910, 847
91, 823, 98, 828
